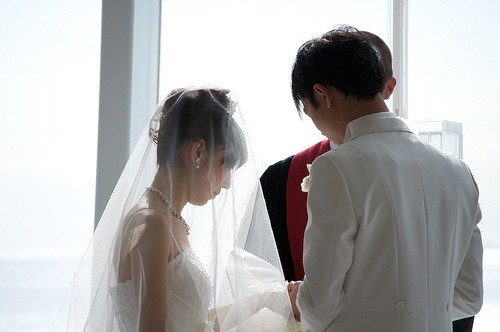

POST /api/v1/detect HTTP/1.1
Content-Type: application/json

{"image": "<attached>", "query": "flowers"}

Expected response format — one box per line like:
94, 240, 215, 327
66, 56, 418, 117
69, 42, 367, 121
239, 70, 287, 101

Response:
301, 164, 312, 192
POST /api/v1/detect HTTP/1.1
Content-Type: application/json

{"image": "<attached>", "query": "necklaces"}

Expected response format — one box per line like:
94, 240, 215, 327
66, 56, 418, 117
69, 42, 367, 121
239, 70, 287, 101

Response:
146, 185, 191, 235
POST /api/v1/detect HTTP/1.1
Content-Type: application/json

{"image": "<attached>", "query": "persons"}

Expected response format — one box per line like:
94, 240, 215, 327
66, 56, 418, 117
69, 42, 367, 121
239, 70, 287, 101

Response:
284, 29, 483, 332
218, 30, 476, 331
56, 86, 297, 332
